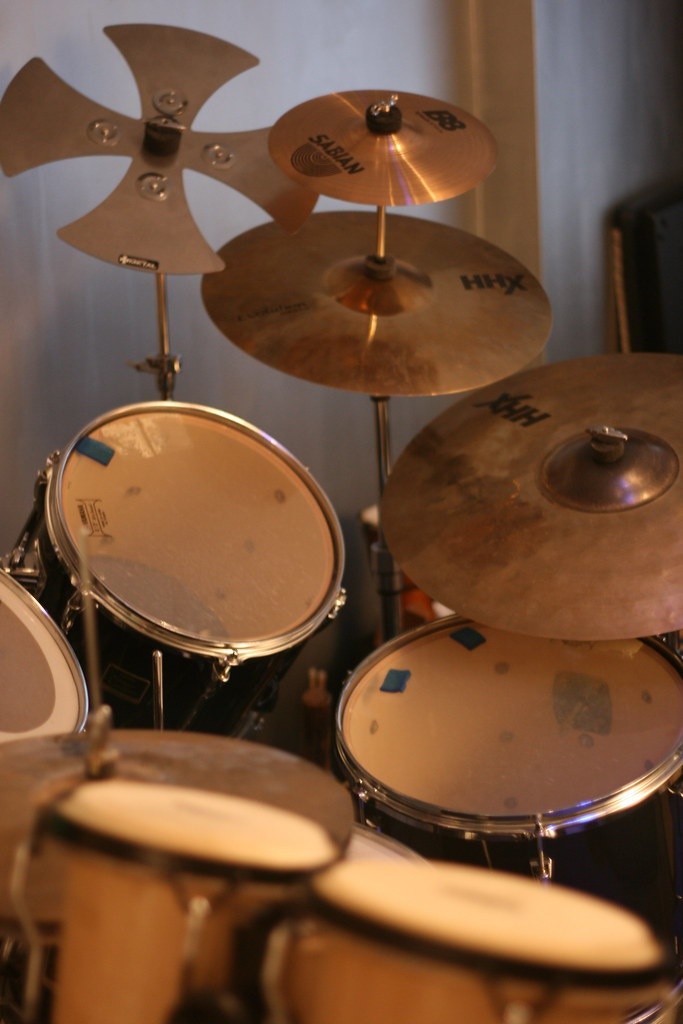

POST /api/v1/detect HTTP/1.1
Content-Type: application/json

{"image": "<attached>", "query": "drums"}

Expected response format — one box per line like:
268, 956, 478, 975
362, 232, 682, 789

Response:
0, 398, 348, 741
39, 776, 341, 1023
332, 611, 682, 964
0, 565, 90, 741
279, 855, 681, 1023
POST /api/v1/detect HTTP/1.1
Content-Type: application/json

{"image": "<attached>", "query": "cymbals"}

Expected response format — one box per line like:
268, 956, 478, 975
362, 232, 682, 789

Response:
200, 209, 555, 398
0, 727, 355, 946
378, 351, 683, 645
268, 85, 504, 208
